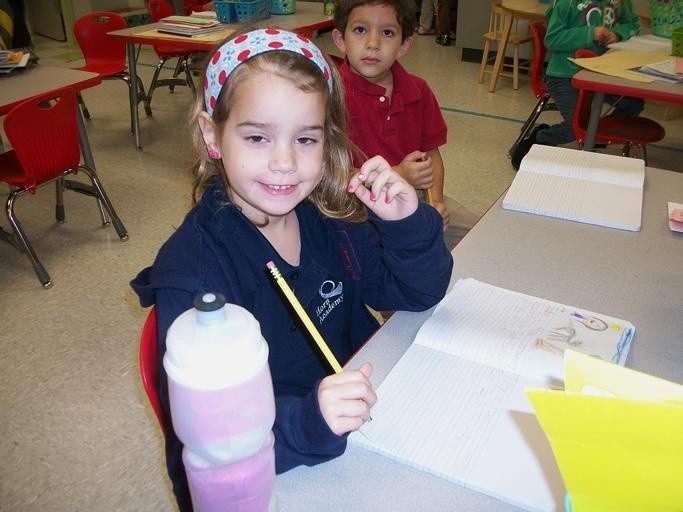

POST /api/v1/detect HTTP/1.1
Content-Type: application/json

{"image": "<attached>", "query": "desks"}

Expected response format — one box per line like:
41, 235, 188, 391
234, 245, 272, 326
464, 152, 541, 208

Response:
270, 165, 683, 512
106, 0, 336, 150
486, 0, 552, 93
570, 28, 683, 167
0, 65, 111, 255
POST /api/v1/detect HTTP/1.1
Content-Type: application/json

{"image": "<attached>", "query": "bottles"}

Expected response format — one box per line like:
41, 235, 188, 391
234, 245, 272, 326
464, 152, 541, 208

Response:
163, 292, 279, 512
323, 1, 337, 16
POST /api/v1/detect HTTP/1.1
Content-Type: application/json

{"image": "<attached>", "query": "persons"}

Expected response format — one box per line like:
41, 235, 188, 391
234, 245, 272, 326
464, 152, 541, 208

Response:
328, 1, 473, 251
125, 23, 454, 511
510, 1, 644, 172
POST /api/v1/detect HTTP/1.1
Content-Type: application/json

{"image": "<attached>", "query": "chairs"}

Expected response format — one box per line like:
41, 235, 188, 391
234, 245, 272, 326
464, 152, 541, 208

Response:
145, 0, 208, 107
68, 10, 152, 136
477, 3, 536, 90
507, 20, 585, 159
572, 48, 665, 167
139, 306, 174, 431
0, 84, 129, 289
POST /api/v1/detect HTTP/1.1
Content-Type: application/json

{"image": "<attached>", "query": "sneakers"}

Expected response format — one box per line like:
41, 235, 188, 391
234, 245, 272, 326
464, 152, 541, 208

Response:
512, 124, 550, 171
413, 25, 456, 46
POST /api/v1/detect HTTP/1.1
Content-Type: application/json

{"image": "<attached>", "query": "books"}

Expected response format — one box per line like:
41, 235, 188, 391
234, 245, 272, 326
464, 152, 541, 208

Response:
627, 57, 682, 85
606, 31, 671, 55
501, 144, 648, 233
348, 277, 636, 512
0, 48, 32, 78
157, 14, 225, 45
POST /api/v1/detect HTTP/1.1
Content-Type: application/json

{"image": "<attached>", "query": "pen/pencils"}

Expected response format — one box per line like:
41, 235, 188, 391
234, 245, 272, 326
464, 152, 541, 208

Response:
266, 261, 373, 420
157, 30, 192, 38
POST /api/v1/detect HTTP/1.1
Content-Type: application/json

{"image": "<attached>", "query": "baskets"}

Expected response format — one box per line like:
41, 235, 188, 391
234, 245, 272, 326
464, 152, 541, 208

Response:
650, 0, 683, 57
214, 1, 296, 24
